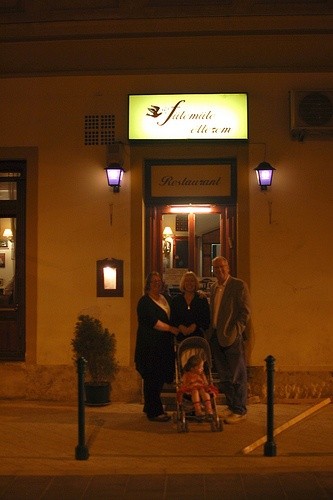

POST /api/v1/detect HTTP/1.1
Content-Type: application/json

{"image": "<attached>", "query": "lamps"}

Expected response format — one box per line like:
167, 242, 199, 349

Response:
254, 161, 277, 191
2, 228, 14, 243
103, 161, 125, 193
162, 226, 174, 254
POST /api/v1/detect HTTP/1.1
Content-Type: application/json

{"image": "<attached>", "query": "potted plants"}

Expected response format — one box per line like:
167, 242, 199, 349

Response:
70, 313, 120, 403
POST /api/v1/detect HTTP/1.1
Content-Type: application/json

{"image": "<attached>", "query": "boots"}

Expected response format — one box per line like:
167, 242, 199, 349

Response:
193, 402, 205, 416
204, 400, 215, 416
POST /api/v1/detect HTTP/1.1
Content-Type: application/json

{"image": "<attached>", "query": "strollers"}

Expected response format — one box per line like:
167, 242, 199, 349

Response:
173, 333, 224, 432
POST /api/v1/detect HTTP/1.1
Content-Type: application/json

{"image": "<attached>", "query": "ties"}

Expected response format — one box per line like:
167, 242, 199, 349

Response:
213, 285, 223, 327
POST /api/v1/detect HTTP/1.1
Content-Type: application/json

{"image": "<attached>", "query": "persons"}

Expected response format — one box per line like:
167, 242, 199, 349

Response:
209, 256, 250, 424
136, 272, 206, 420
181, 354, 215, 417
170, 271, 211, 423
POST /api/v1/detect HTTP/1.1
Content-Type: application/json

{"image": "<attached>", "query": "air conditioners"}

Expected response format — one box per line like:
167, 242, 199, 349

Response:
288, 87, 333, 135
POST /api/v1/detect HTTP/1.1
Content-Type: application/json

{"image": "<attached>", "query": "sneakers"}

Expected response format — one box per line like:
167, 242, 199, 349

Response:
218, 408, 234, 417
225, 413, 249, 424
151, 413, 171, 422
